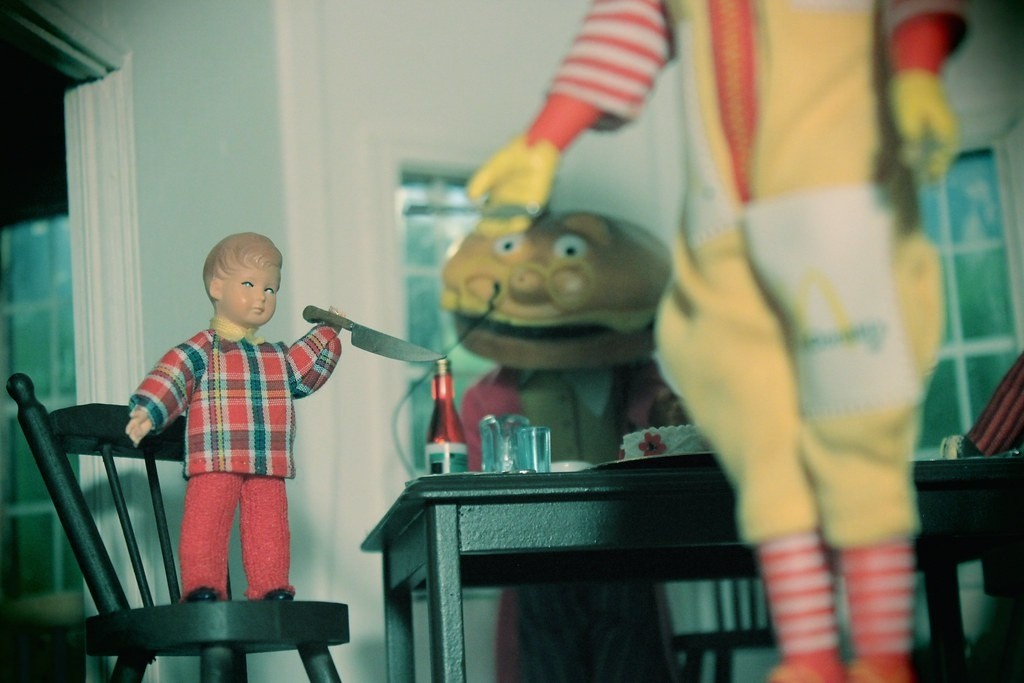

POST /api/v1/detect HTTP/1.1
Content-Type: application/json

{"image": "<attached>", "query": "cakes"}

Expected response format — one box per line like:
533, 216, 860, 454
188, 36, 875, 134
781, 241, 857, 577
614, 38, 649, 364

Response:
619, 423, 709, 459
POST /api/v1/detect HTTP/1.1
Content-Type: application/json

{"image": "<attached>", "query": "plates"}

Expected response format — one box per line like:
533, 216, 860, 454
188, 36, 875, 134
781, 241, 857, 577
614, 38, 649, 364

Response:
622, 425, 708, 458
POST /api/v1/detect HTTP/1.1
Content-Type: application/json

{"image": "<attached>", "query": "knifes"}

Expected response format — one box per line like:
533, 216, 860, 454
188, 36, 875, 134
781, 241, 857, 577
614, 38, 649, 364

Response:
303, 305, 447, 362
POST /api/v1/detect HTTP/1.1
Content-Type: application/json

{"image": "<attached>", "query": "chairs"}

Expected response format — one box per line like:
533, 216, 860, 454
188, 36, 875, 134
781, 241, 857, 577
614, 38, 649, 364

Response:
672, 580, 777, 683
6, 372, 350, 683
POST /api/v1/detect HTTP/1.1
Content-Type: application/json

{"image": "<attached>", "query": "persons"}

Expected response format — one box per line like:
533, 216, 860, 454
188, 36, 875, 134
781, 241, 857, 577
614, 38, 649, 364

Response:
125, 232, 343, 601
442, 0, 969, 683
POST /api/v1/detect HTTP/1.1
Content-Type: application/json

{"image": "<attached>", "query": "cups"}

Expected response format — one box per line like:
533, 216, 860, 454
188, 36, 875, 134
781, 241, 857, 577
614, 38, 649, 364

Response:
477, 415, 530, 472
518, 426, 552, 475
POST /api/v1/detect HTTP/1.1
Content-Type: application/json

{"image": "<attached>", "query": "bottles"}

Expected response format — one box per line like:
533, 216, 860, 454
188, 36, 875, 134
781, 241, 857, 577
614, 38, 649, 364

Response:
425, 359, 469, 474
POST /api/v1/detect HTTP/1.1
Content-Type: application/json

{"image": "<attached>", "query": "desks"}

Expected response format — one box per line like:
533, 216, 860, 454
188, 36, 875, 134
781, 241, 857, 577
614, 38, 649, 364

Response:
361, 453, 1024, 683
